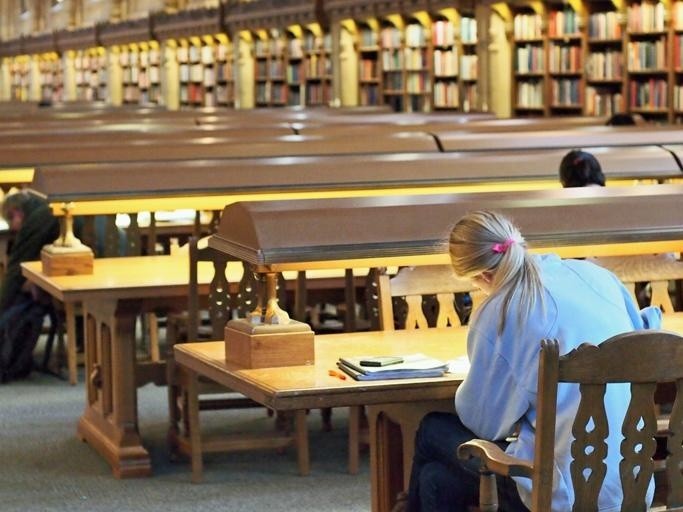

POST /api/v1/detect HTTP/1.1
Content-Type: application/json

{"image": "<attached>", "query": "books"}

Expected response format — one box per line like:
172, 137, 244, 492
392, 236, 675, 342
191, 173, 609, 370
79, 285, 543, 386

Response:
335, 353, 450, 381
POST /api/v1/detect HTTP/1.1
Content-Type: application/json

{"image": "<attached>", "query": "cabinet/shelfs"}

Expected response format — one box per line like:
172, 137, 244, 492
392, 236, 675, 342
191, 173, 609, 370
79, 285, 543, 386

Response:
235, 1, 682, 124
0, 0, 234, 110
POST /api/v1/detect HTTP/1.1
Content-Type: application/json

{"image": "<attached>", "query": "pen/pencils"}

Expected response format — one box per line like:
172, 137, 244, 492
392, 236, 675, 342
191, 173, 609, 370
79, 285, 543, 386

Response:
329, 369, 346, 380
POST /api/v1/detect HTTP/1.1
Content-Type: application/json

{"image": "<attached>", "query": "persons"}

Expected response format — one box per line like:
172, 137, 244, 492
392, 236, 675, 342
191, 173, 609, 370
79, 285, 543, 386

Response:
0, 191, 125, 380
558, 150, 607, 187
404, 208, 664, 511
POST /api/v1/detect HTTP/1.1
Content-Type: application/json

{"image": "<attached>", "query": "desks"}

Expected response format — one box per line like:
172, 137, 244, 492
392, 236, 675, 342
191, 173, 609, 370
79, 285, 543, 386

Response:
16, 242, 399, 484
173, 311, 683, 512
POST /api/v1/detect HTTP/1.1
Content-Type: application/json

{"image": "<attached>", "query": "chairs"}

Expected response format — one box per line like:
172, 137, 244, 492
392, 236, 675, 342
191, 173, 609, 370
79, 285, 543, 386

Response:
189, 209, 354, 341
377, 264, 483, 330
457, 330, 682, 512
587, 251, 682, 313
